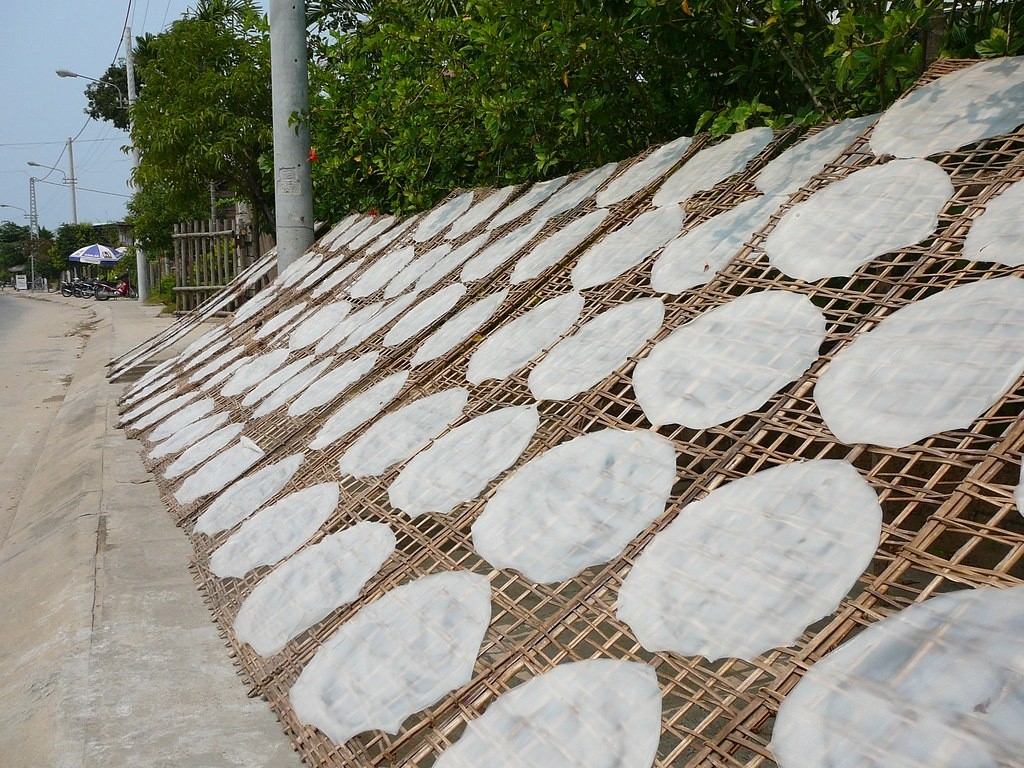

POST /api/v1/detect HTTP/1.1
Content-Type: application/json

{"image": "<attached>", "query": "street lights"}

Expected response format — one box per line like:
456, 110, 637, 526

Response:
27, 161, 79, 278
56, 68, 147, 301
0, 204, 35, 290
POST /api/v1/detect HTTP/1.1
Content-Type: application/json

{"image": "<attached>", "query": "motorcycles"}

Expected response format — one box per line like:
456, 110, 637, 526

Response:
61, 269, 133, 302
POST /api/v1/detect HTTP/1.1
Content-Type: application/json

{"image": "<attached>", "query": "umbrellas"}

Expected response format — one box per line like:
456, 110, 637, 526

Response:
69, 243, 126, 281
